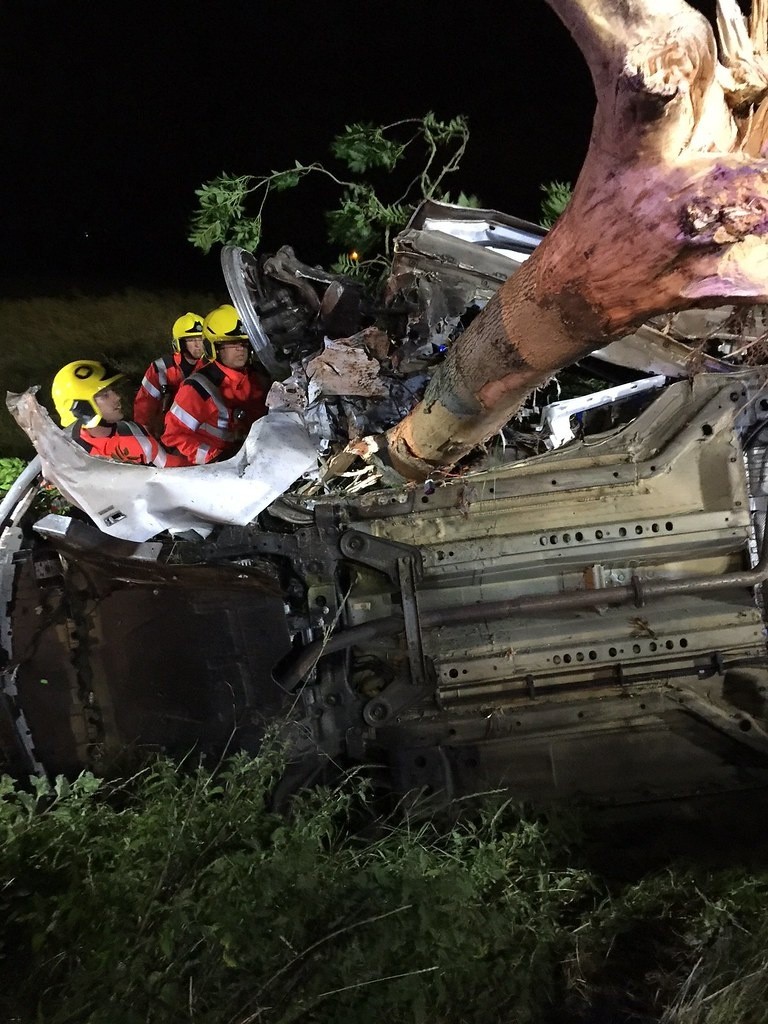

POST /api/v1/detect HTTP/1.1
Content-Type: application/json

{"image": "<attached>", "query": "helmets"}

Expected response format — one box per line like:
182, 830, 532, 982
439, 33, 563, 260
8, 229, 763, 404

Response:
203, 305, 249, 362
51, 359, 126, 430
172, 312, 205, 352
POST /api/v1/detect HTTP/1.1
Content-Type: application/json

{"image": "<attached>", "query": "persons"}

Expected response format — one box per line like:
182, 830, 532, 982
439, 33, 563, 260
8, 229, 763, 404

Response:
51, 360, 196, 467
133, 313, 205, 454
160, 305, 269, 465
